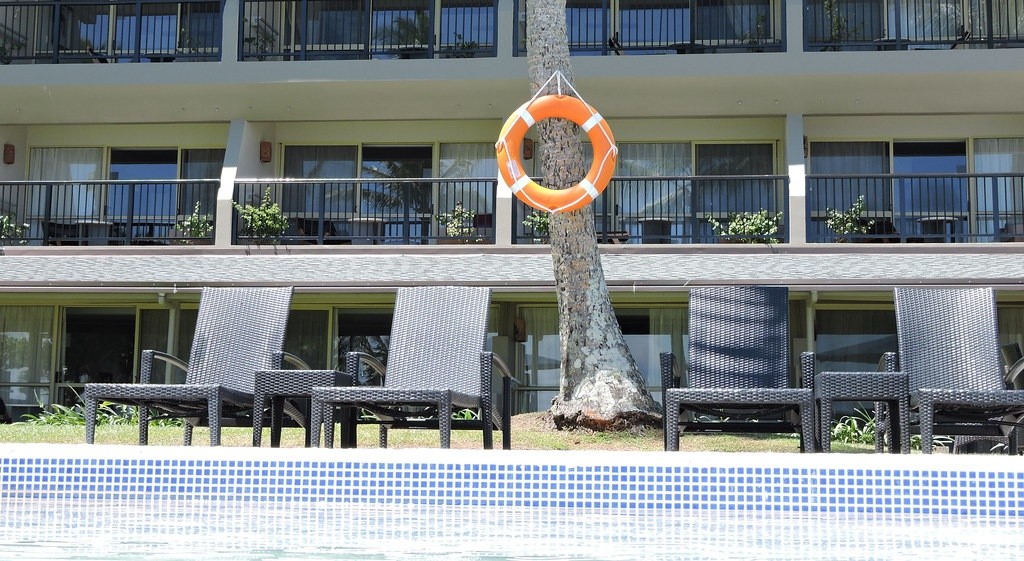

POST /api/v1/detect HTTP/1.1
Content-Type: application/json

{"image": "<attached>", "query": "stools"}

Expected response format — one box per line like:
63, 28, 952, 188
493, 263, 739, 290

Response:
917, 217, 958, 242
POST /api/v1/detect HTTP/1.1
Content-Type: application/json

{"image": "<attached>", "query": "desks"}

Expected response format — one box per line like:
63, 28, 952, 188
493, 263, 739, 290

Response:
251, 369, 352, 449
145, 53, 175, 63
348, 218, 387, 246
814, 372, 909, 454
872, 38, 911, 51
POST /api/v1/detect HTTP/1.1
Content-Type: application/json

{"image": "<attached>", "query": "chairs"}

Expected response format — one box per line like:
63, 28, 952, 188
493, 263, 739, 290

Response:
891, 286, 1024, 456
660, 286, 816, 453
609, 32, 626, 56
915, 26, 973, 50
311, 286, 511, 450
87, 46, 109, 64
85, 283, 294, 445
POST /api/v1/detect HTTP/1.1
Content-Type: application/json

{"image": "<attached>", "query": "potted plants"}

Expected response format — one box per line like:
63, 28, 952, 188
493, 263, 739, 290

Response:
377, 13, 429, 58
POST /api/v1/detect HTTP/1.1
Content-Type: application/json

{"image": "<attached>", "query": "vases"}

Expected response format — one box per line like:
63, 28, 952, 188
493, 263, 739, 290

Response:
250, 231, 272, 245
188, 239, 211, 245
369, 48, 396, 59
721, 237, 731, 244
174, 200, 213, 237
833, 237, 847, 243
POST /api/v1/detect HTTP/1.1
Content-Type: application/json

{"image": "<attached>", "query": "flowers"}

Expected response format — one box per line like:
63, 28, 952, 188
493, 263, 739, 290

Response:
825, 195, 874, 235
521, 211, 548, 241
436, 201, 474, 237
233, 188, 289, 243
704, 208, 783, 243
0, 216, 30, 246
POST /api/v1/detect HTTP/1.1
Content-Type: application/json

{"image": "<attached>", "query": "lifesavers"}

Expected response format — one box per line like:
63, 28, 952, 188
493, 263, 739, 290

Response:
493, 90, 621, 214
